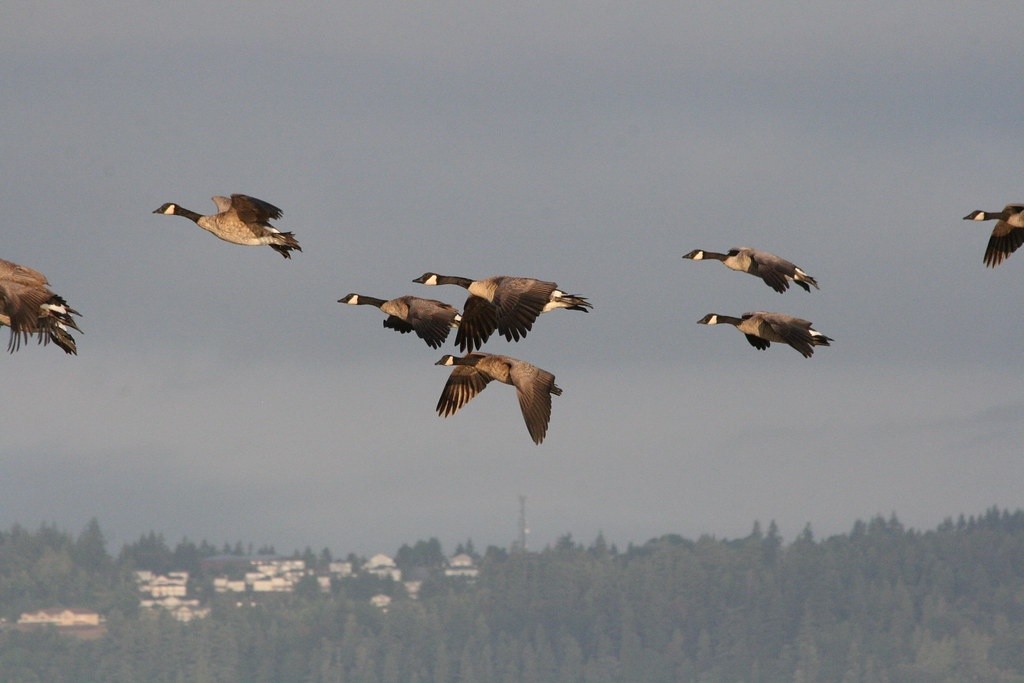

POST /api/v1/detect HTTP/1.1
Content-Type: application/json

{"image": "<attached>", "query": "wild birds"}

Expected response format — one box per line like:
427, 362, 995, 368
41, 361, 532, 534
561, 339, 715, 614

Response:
696, 311, 834, 359
412, 271, 594, 354
434, 352, 563, 445
0, 257, 83, 355
682, 246, 821, 295
337, 292, 463, 350
153, 193, 304, 259
962, 202, 1024, 268
